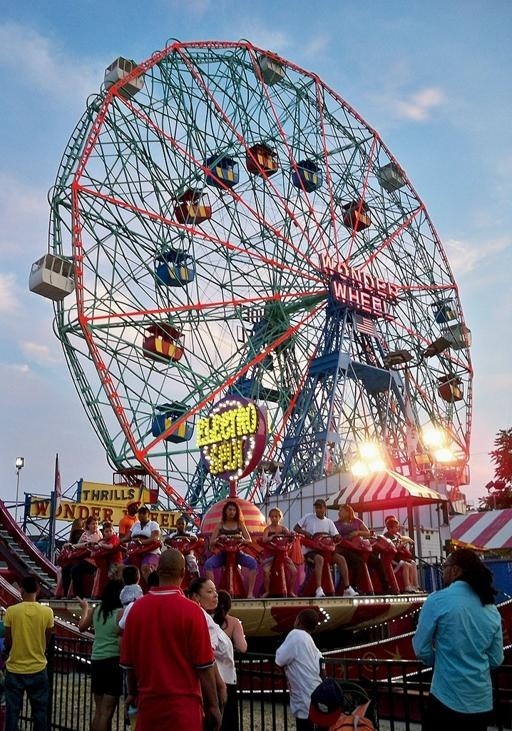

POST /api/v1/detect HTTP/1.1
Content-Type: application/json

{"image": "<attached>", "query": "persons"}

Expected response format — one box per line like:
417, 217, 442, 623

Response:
273, 607, 327, 731
2, 576, 57, 731
76, 549, 248, 730
55, 496, 423, 598
411, 547, 505, 731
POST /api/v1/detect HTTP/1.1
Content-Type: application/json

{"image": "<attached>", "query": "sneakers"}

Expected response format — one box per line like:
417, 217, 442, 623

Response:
248, 585, 427, 598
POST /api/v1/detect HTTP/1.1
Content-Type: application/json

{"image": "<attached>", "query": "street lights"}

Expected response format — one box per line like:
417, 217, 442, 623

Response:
10, 455, 29, 522
483, 479, 509, 507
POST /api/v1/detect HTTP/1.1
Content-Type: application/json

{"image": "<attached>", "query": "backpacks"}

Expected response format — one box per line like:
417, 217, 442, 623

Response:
329, 700, 378, 731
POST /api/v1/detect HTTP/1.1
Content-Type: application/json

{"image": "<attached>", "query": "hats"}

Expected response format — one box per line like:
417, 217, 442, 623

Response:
309, 678, 345, 727
313, 499, 325, 506
127, 499, 141, 508
